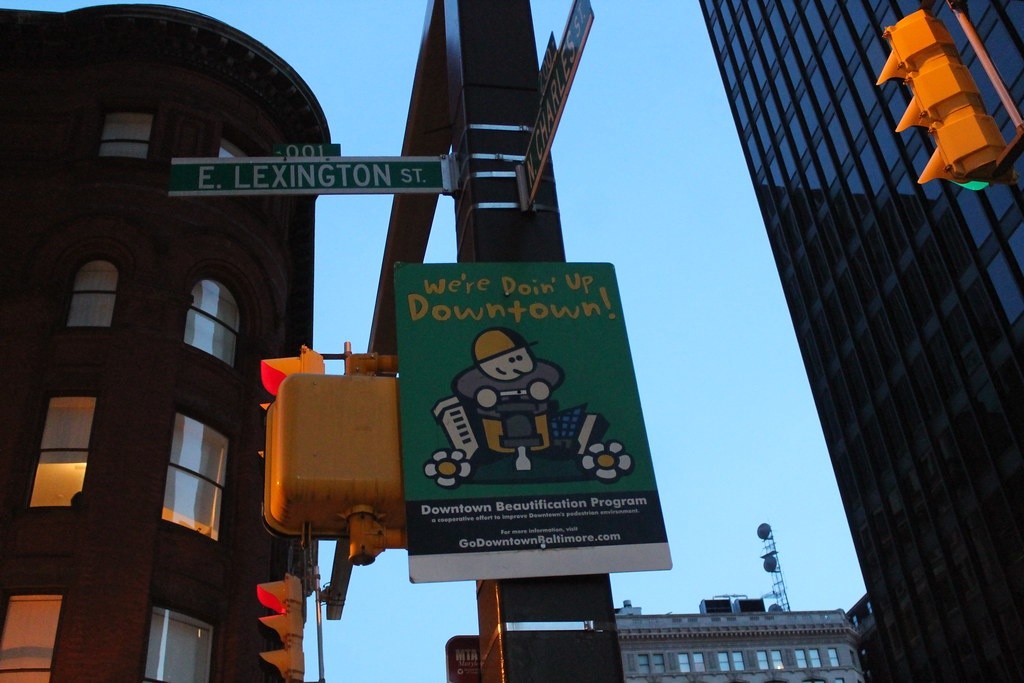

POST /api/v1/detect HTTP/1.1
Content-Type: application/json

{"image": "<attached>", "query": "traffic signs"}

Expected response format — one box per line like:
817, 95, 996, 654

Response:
166, 142, 452, 198
517, 0, 596, 209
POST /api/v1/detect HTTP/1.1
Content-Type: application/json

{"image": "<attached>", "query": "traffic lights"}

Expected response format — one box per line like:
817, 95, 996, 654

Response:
256, 573, 303, 683
875, 8, 1017, 191
260, 345, 326, 398
